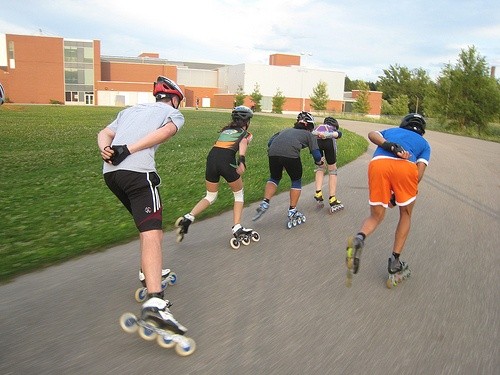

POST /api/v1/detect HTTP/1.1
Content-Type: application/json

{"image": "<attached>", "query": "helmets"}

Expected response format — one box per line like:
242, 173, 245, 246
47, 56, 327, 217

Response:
153, 75, 184, 100
324, 116, 339, 131
399, 113, 426, 134
296, 111, 315, 124
231, 105, 253, 120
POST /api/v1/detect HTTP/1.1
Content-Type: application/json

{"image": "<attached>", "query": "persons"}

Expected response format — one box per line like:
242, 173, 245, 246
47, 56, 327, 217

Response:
312, 117, 345, 213
345, 113, 430, 289
98, 76, 196, 357
175, 105, 260, 249
253, 112, 325, 228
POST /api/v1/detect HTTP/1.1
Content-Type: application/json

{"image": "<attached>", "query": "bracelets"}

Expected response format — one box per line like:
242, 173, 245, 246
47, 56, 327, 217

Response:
104, 146, 111, 150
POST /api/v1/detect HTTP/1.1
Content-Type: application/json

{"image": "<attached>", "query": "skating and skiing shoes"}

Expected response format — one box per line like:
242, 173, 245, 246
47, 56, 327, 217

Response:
230, 223, 260, 249
387, 256, 411, 288
345, 235, 364, 288
120, 297, 196, 356
329, 196, 344, 215
135, 268, 177, 303
286, 207, 306, 229
175, 213, 195, 243
314, 190, 325, 209
252, 200, 271, 222
